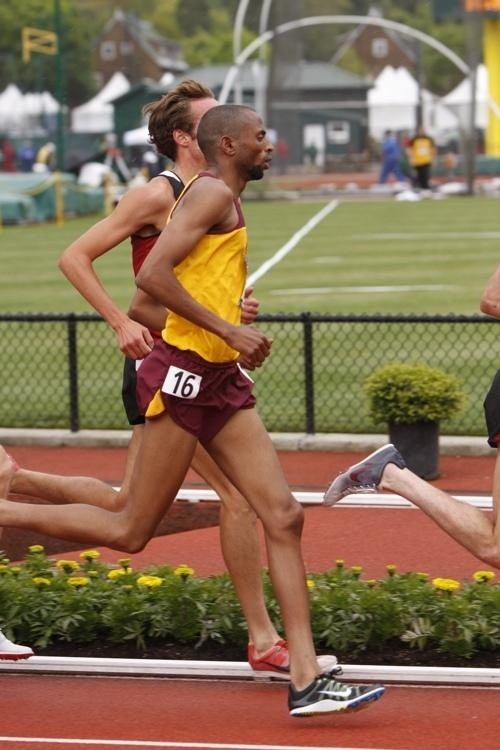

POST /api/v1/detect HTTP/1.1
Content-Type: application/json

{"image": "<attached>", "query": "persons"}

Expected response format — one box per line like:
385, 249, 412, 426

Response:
323, 260, 499, 575
305, 142, 318, 167
1, 134, 57, 172
0, 76, 345, 680
276, 137, 289, 175
0, 630, 39, 662
0, 104, 391, 721
380, 126, 437, 190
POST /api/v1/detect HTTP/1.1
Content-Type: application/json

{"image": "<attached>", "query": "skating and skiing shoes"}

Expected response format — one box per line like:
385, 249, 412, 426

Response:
0, 629, 33, 661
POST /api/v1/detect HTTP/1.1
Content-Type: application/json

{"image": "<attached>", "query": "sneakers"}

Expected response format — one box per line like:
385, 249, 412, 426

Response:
286, 678, 386, 717
246, 636, 338, 679
321, 444, 407, 508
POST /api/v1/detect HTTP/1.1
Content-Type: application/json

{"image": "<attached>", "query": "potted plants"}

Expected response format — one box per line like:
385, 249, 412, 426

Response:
366, 362, 467, 482
